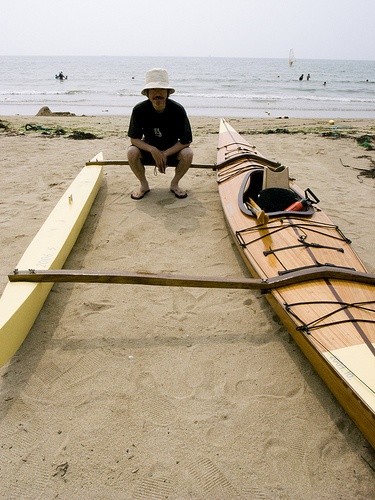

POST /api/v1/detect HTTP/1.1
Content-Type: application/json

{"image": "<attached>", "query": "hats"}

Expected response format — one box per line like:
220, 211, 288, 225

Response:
141, 68, 175, 95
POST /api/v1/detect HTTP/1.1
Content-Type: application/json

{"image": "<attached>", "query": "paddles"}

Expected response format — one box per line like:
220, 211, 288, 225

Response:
245, 196, 269, 225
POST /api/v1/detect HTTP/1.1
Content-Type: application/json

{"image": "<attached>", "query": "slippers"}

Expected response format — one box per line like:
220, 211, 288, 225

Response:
170, 188, 187, 199
131, 189, 150, 200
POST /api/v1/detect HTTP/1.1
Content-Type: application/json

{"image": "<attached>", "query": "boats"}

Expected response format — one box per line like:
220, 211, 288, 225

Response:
0, 117, 375, 451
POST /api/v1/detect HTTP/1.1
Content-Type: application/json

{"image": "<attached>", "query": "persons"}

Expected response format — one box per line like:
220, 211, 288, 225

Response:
307, 74, 310, 80
299, 73, 304, 81
127, 68, 194, 200
